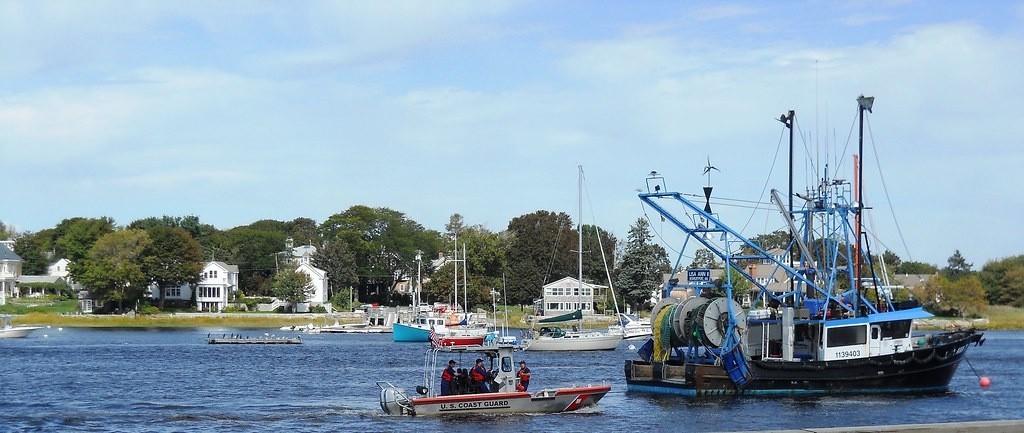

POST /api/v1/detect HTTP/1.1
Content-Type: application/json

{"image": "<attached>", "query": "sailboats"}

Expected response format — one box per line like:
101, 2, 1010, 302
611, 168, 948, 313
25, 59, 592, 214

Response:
518, 164, 628, 353
390, 231, 493, 341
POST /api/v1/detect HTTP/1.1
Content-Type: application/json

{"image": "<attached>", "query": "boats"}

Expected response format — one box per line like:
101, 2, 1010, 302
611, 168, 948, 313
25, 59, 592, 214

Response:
206, 334, 304, 345
318, 301, 433, 333
622, 91, 992, 396
1, 323, 44, 338
372, 326, 613, 419
428, 320, 500, 354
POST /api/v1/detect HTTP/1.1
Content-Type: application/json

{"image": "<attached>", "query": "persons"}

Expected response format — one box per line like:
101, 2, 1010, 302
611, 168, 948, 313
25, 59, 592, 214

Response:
516, 360, 531, 392
443, 359, 465, 393
470, 358, 494, 393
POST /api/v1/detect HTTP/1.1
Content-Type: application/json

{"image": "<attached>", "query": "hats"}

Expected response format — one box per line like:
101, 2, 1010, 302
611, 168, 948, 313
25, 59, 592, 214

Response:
449, 360, 456, 363
519, 361, 525, 364
476, 359, 483, 364
